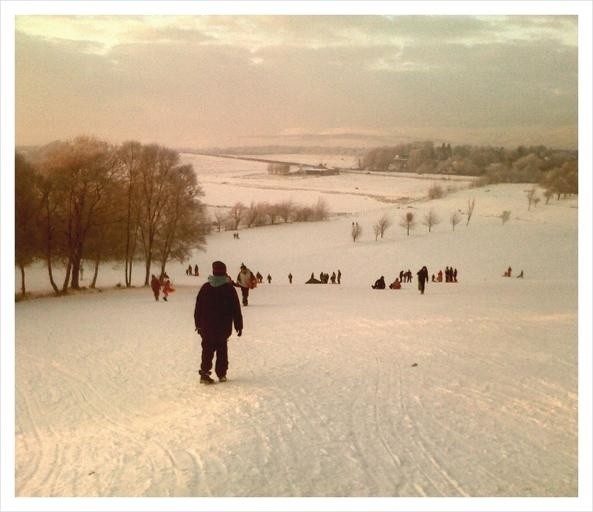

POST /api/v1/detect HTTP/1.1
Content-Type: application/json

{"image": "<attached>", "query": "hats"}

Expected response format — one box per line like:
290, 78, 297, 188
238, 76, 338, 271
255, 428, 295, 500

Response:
212, 261, 226, 276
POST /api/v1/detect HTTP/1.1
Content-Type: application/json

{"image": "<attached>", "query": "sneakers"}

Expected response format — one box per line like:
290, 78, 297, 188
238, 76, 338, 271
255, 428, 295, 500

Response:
218, 376, 228, 382
200, 375, 215, 384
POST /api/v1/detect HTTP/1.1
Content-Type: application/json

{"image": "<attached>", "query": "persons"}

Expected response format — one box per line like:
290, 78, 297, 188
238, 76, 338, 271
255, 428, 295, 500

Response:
147, 259, 262, 307
266, 273, 271, 283
304, 270, 341, 284
193, 261, 242, 385
415, 265, 429, 294
287, 273, 292, 283
370, 269, 412, 289
431, 266, 461, 283
